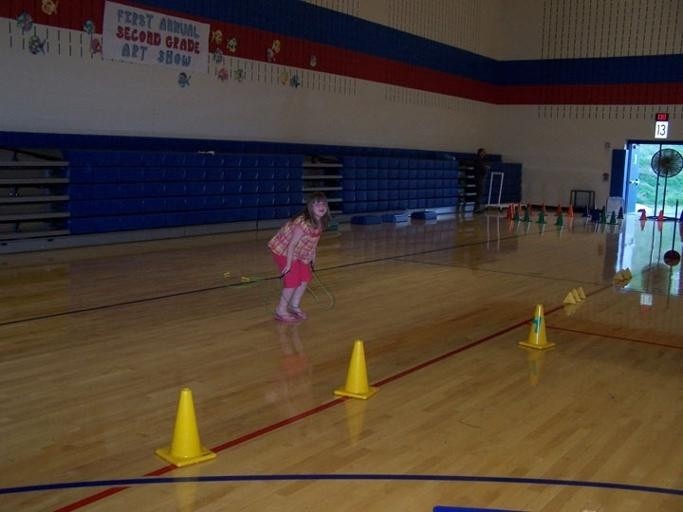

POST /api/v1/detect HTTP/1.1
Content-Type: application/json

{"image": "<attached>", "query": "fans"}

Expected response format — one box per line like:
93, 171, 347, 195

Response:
650, 149, 683, 219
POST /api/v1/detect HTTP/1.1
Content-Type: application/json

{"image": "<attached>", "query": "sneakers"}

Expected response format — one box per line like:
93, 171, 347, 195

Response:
272, 307, 308, 322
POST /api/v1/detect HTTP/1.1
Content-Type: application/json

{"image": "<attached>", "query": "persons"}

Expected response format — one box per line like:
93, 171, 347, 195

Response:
266, 192, 333, 321
472, 148, 490, 212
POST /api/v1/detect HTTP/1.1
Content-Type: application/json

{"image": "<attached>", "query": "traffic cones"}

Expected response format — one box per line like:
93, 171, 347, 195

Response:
333, 339, 380, 400
639, 209, 646, 220
517, 303, 555, 349
657, 210, 663, 220
678, 210, 683, 221
554, 203, 575, 226
507, 219, 683, 236
507, 203, 547, 223
153, 388, 216, 467
581, 200, 624, 225
